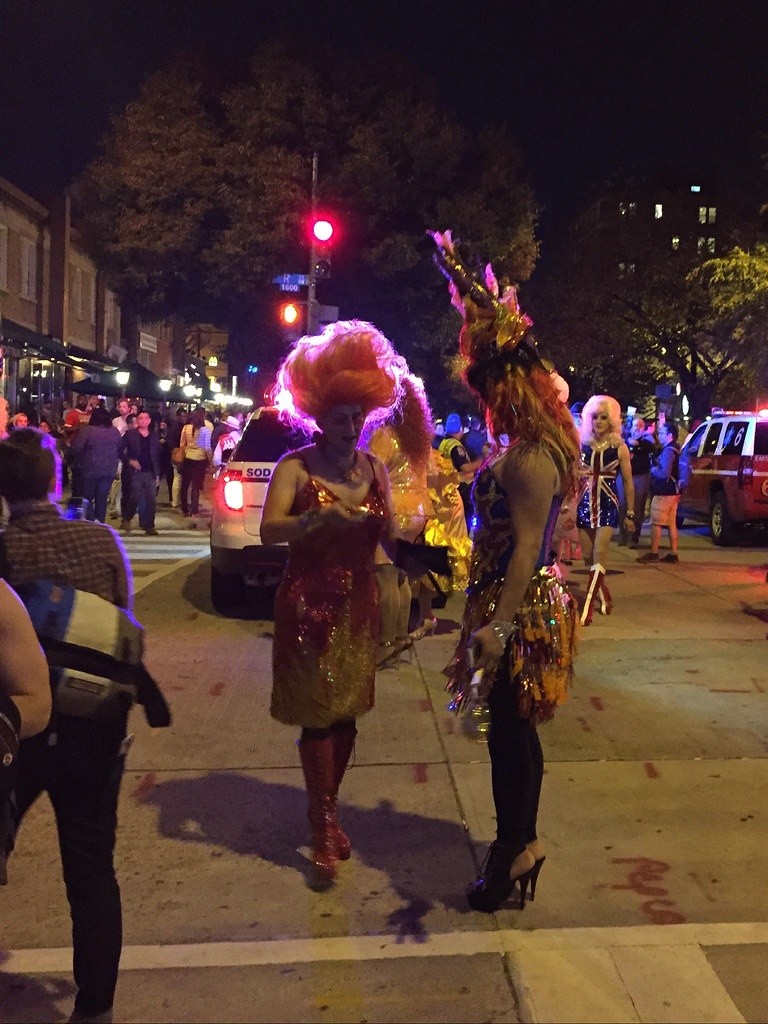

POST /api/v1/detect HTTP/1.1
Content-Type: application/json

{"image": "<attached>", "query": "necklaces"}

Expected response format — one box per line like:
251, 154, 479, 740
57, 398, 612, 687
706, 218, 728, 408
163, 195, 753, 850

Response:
311, 430, 363, 485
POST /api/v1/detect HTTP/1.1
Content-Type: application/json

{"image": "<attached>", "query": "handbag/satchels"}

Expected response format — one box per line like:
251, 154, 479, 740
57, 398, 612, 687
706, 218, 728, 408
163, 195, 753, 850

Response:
170, 423, 187, 469
12, 582, 145, 732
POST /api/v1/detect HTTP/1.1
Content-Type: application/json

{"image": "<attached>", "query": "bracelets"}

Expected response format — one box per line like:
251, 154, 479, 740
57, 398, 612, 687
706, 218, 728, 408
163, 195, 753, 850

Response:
626, 510, 635, 521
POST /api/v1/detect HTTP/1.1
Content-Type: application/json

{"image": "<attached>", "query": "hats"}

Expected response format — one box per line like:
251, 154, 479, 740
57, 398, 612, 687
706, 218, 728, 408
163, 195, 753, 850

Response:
445, 413, 462, 433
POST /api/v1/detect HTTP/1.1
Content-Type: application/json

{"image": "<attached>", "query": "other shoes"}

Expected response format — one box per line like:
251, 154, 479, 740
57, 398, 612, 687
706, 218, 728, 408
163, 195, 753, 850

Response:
145, 527, 158, 535
118, 520, 129, 531
617, 534, 638, 549
636, 553, 659, 563
659, 553, 681, 564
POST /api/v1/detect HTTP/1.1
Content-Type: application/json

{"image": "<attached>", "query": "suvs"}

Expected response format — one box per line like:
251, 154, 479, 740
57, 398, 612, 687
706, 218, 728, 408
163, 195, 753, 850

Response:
208, 405, 325, 618
676, 406, 768, 546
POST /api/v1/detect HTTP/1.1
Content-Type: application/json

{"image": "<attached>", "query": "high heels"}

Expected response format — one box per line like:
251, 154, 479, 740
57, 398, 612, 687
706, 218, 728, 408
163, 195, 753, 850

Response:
373, 635, 414, 670
467, 837, 547, 912
408, 616, 438, 641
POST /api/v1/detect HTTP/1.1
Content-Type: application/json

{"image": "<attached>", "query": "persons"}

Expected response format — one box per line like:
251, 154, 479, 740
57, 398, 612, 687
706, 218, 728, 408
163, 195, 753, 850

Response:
0, 385, 253, 536
366, 371, 703, 683
428, 229, 583, 913
260, 319, 409, 880
0, 428, 175, 1024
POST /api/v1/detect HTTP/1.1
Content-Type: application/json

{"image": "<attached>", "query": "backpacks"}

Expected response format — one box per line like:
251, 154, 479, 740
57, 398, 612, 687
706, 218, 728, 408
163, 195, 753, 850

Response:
661, 446, 689, 489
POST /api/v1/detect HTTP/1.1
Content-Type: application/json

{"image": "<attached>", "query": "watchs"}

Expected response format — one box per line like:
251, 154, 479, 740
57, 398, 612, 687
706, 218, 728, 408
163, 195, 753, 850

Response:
489, 620, 506, 648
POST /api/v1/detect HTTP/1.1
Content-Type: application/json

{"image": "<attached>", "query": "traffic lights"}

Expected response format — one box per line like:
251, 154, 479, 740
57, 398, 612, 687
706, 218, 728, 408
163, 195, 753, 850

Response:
758, 408, 768, 418
278, 303, 303, 329
309, 219, 335, 280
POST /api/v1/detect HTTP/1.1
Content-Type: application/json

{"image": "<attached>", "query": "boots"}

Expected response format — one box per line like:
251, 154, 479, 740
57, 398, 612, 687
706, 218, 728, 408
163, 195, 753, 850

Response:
295, 729, 357, 892
579, 563, 611, 627
